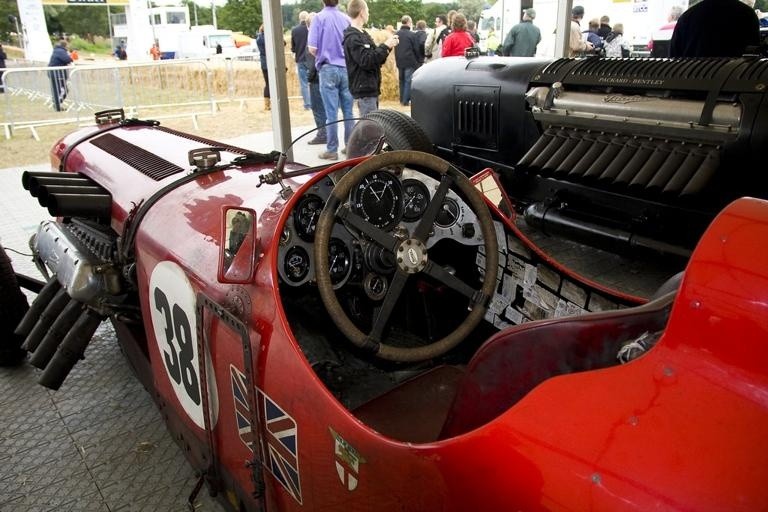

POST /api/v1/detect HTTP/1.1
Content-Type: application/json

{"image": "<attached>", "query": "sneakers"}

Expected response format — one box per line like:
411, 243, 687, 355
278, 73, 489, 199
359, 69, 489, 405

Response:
319, 151, 338, 160
264, 97, 272, 111
54, 106, 67, 112
341, 144, 347, 154
308, 132, 327, 144
402, 101, 411, 106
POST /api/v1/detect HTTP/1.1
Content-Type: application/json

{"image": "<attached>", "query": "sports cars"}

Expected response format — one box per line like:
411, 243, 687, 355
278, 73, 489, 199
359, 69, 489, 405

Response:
408, 46, 768, 268
13, 108, 768, 512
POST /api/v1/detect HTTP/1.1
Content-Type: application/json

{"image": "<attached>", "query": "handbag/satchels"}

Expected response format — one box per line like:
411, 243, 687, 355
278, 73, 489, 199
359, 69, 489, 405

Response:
425, 28, 437, 57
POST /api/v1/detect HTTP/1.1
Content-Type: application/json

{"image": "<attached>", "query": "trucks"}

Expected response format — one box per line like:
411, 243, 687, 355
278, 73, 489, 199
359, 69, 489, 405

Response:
196, 28, 246, 62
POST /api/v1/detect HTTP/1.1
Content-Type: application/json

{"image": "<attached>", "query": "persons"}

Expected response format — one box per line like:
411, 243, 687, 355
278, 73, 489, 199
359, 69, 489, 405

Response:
342, 0, 393, 126
214, 42, 223, 54
113, 44, 129, 62
394, 12, 482, 105
309, 1, 357, 163
256, 23, 289, 112
564, 7, 630, 63
47, 41, 75, 111
150, 44, 161, 62
649, 6, 685, 52
290, 11, 320, 106
1, 45, 8, 92
497, 8, 545, 59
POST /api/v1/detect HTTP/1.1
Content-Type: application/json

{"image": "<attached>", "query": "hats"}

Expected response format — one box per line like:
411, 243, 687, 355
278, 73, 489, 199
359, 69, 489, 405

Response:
523, 8, 536, 20
572, 6, 584, 16
